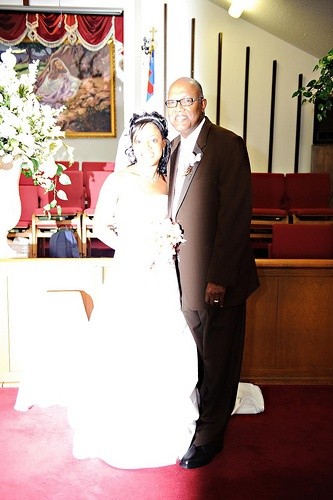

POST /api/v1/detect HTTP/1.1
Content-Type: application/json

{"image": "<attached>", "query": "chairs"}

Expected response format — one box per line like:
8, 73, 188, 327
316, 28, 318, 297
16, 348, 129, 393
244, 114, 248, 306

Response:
251, 173, 333, 259
6, 162, 115, 260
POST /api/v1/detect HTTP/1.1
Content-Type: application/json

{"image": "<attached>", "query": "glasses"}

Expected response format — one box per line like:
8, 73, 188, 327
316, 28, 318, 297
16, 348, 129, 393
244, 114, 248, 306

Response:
165, 97, 202, 108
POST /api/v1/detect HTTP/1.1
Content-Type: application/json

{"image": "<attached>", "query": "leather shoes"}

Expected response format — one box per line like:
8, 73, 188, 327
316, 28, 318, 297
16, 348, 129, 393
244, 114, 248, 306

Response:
180, 439, 225, 469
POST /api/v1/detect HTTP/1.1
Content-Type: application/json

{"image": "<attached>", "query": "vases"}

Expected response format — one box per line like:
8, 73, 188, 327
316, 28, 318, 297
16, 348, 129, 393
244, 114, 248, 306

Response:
0, 166, 22, 231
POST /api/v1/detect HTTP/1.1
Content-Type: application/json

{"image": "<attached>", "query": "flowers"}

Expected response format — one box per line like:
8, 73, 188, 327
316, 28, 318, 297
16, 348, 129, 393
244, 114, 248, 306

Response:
0, 50, 74, 200
185, 152, 201, 176
150, 218, 185, 264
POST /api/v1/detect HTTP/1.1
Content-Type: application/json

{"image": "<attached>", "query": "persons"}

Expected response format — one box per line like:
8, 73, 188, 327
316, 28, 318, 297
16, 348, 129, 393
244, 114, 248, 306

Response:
70, 111, 200, 470
153, 76, 261, 468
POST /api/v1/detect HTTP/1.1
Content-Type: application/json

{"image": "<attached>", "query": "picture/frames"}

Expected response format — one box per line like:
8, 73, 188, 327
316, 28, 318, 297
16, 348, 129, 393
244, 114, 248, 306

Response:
0, 38, 116, 138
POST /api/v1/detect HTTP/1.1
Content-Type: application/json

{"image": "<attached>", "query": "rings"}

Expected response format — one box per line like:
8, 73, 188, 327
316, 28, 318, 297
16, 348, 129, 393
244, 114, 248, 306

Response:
214, 300, 219, 303
